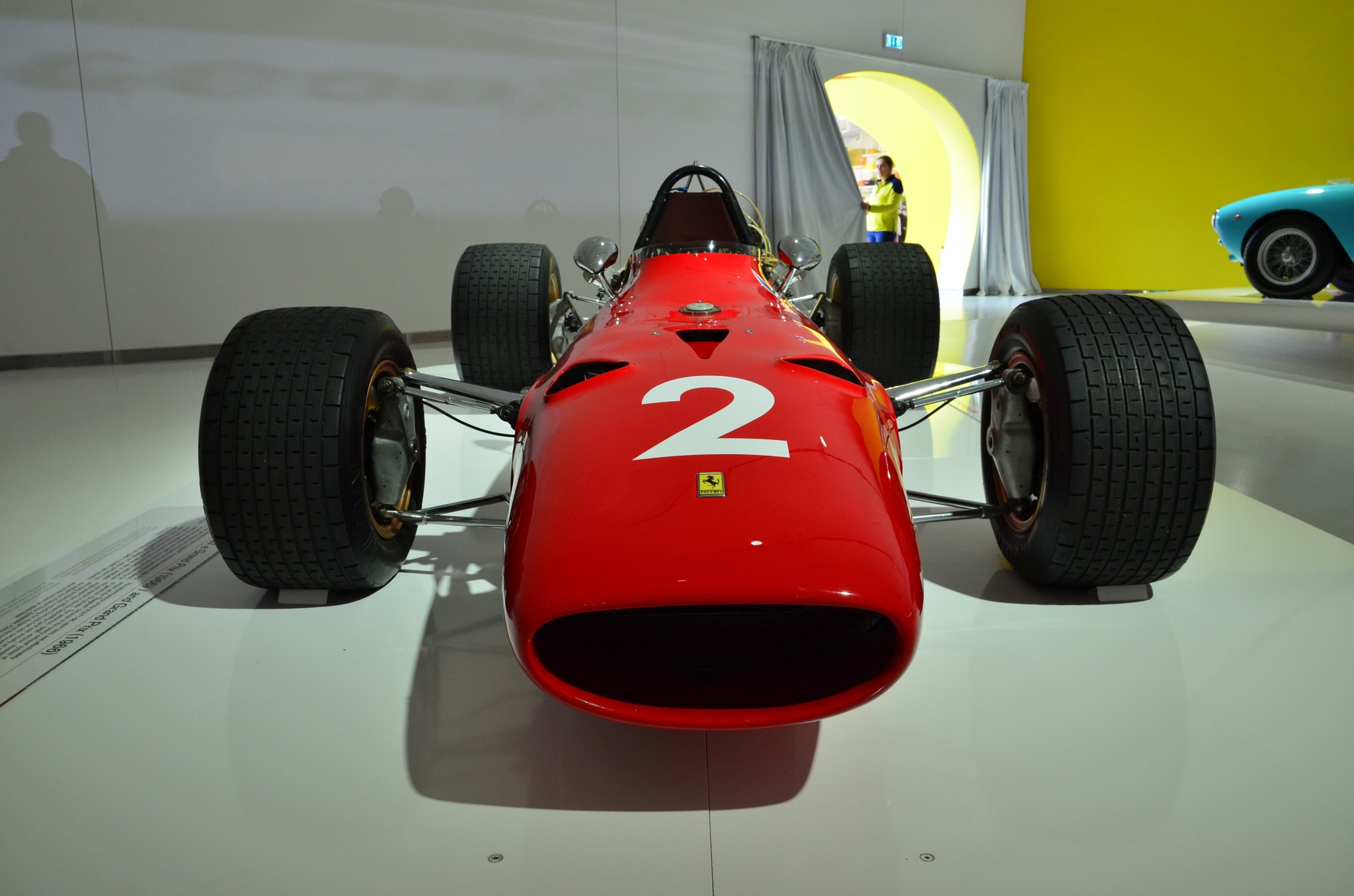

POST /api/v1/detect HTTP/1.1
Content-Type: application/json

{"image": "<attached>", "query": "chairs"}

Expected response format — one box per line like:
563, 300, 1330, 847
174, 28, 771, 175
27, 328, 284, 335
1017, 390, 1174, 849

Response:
632, 164, 762, 263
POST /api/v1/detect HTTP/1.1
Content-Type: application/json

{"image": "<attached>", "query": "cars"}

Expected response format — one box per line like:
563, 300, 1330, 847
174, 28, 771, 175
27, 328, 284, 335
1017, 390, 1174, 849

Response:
1212, 182, 1354, 296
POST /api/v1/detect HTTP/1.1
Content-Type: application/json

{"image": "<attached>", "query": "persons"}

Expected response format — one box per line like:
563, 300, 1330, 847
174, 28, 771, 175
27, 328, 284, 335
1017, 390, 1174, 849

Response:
861, 156, 903, 244
